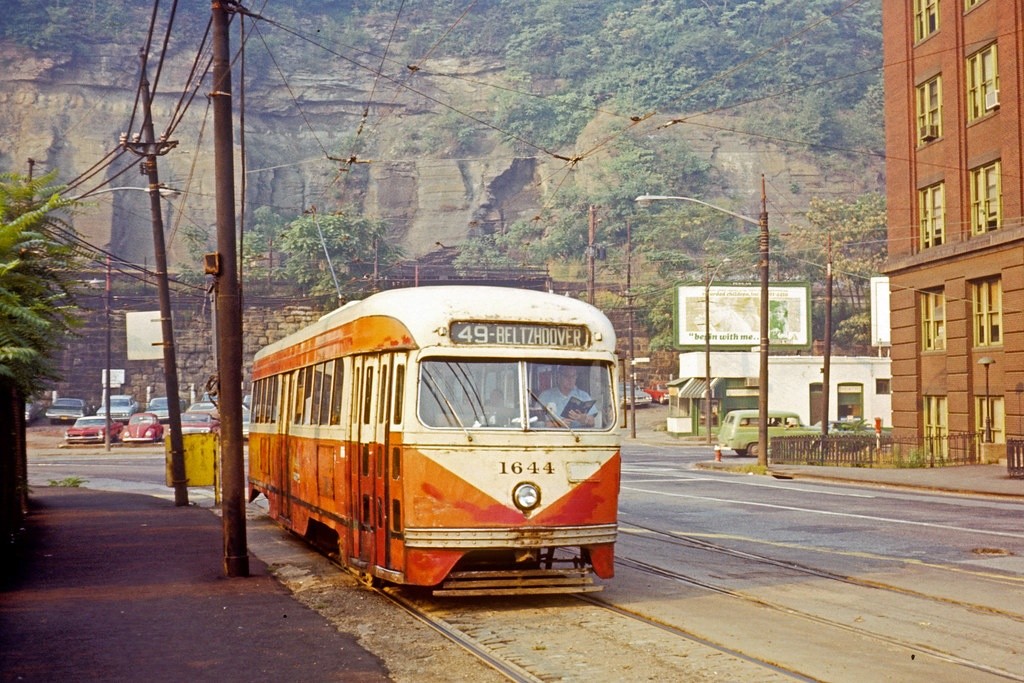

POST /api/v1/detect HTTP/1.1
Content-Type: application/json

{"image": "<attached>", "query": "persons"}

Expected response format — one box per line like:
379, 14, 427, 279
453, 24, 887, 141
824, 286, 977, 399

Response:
533, 364, 598, 428
773, 418, 792, 427
769, 300, 789, 339
490, 390, 504, 408
694, 298, 752, 333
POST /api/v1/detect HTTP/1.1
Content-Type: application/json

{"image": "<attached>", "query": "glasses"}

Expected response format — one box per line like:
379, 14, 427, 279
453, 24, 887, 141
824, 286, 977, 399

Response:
560, 371, 577, 379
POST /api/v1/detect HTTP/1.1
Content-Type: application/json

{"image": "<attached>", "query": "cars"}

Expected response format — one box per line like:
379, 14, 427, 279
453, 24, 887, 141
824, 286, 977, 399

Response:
242, 405, 251, 441
45, 398, 90, 424
201, 391, 218, 403
120, 412, 164, 444
180, 412, 221, 441
187, 403, 220, 419
64, 416, 123, 445
618, 381, 652, 409
716, 409, 838, 458
25, 402, 44, 426
145, 397, 169, 421
96, 394, 140, 425
642, 380, 669, 404
242, 395, 253, 409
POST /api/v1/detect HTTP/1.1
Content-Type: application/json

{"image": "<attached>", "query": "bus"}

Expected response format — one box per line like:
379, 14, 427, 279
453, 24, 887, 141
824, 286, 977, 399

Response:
247, 284, 623, 599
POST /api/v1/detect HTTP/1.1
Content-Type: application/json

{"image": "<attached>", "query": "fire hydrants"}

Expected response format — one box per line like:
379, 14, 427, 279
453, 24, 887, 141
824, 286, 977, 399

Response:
714, 445, 723, 462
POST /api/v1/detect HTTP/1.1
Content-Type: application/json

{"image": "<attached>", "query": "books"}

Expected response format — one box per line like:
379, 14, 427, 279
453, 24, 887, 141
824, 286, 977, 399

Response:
561, 396, 597, 420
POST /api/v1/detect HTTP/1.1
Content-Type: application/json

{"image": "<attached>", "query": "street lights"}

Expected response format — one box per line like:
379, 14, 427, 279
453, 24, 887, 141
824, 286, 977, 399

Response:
704, 259, 733, 443
635, 194, 768, 465
23, 185, 182, 240
